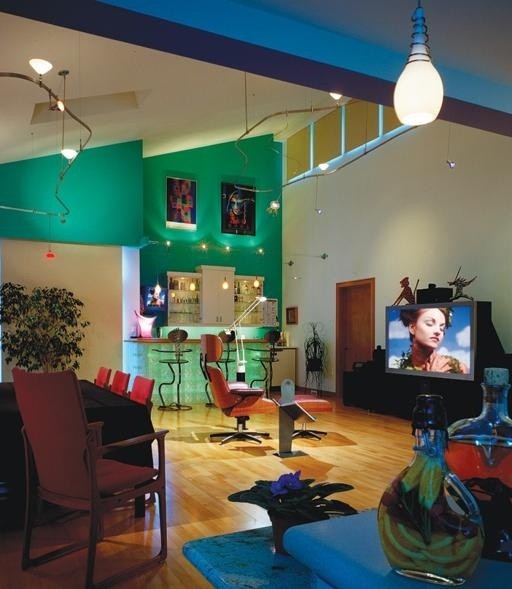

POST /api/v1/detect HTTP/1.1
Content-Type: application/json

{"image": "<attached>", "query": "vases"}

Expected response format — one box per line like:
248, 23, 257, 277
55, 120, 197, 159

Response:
270, 518, 307, 555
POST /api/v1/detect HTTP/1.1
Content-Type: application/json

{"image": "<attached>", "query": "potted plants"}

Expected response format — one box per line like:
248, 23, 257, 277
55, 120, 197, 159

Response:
303, 321, 328, 397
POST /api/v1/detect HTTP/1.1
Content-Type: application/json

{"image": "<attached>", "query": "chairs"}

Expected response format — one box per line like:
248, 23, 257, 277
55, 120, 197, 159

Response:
152, 329, 195, 411
250, 330, 282, 397
200, 335, 276, 445
10, 367, 169, 588
93, 367, 154, 405
198, 329, 238, 407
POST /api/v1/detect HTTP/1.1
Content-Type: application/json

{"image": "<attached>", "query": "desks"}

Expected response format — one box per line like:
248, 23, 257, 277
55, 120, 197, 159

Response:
182, 508, 512, 589
0, 379, 155, 538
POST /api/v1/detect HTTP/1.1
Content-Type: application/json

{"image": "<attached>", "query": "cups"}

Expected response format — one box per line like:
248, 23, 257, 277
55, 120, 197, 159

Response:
173, 293, 198, 304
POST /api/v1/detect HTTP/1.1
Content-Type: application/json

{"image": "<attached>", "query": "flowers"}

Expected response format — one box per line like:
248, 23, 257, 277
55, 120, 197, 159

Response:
228, 470, 359, 522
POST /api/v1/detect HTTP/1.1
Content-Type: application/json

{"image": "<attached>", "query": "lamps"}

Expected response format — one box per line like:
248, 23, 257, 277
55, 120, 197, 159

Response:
254, 276, 260, 288
61, 148, 77, 162
190, 278, 196, 291
225, 296, 267, 430
49, 89, 64, 113
328, 91, 342, 101
394, 0, 444, 126
319, 163, 329, 171
28, 57, 53, 87
268, 199, 281, 213
223, 276, 231, 290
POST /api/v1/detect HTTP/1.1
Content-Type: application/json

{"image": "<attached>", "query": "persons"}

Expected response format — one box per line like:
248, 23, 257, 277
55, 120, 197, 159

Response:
398, 306, 467, 375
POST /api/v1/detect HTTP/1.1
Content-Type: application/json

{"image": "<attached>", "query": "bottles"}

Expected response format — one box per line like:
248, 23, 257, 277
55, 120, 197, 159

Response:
446, 367, 512, 564
377, 394, 483, 585
170, 278, 199, 290
233, 281, 261, 295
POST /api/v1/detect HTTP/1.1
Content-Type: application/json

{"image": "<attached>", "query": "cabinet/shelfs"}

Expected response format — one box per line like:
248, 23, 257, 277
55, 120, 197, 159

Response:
342, 369, 483, 428
165, 265, 278, 325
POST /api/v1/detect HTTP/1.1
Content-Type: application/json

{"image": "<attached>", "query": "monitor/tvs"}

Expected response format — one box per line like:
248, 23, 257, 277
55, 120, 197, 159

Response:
385, 301, 491, 382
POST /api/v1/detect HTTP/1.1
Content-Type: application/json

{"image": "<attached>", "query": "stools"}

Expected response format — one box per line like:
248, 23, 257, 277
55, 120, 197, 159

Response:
292, 392, 333, 441
